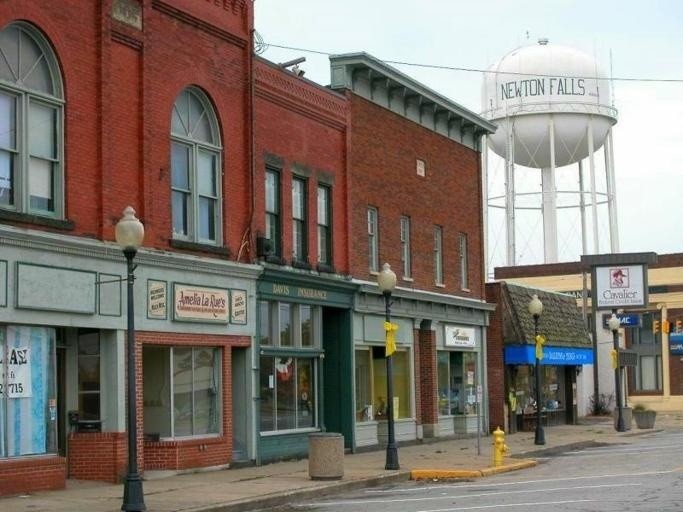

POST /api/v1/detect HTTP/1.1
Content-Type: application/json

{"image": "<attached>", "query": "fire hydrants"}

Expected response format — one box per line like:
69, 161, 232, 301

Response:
492, 426, 509, 466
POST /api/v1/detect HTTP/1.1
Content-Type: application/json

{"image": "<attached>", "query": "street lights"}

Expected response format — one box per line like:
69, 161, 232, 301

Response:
115, 205, 146, 510
528, 295, 545, 445
378, 263, 400, 471
609, 314, 625, 432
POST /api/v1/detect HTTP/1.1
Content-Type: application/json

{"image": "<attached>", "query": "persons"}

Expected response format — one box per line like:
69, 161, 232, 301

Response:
611, 271, 628, 287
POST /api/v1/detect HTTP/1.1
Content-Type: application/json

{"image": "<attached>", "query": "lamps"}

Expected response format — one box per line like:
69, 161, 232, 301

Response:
263, 255, 335, 275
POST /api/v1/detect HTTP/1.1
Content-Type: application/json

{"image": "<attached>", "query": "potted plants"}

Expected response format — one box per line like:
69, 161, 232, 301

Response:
633, 405, 656, 429
